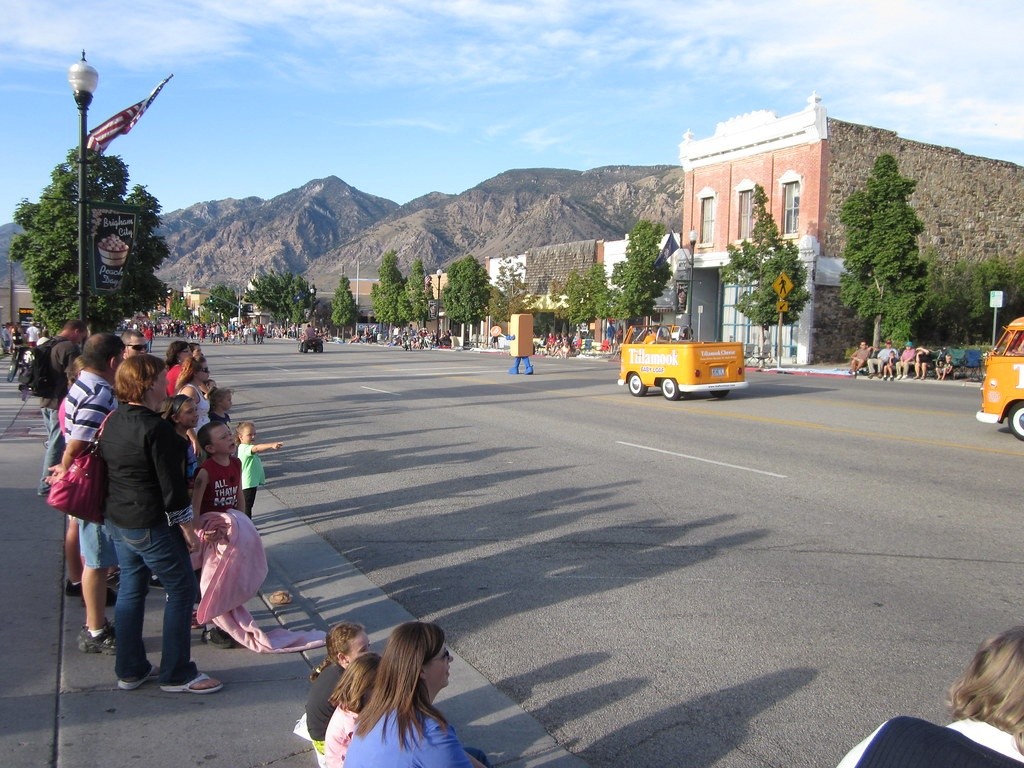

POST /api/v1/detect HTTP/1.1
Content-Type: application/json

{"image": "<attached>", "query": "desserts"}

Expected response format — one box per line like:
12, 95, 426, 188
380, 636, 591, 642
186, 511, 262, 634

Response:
98, 234, 129, 266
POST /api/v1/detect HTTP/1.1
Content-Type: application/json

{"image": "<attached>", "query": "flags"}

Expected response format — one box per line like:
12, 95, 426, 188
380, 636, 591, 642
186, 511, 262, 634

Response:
654, 232, 679, 271
87, 80, 165, 156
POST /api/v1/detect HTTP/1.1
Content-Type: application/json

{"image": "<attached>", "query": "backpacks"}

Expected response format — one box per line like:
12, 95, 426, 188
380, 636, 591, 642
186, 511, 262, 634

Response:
17, 337, 65, 398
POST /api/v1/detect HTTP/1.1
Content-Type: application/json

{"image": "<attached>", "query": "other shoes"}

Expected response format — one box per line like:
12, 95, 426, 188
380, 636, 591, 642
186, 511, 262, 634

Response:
914, 376, 921, 379
884, 375, 909, 381
849, 370, 857, 379
937, 376, 945, 381
190, 608, 204, 630
877, 372, 882, 378
148, 576, 164, 589
107, 564, 123, 590
921, 374, 926, 380
868, 373, 877, 378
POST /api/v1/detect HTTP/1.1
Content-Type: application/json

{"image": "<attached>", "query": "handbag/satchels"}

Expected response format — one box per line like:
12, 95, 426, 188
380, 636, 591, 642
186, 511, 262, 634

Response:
45, 408, 115, 525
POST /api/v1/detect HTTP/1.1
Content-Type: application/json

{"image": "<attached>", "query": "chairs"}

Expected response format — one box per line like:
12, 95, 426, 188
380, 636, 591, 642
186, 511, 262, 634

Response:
938, 349, 982, 381
742, 344, 772, 367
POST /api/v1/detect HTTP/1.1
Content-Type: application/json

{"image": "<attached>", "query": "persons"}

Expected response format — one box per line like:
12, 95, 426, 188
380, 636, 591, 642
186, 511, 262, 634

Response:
163, 341, 283, 648
851, 340, 954, 380
44, 334, 126, 655
680, 328, 694, 340
763, 325, 773, 363
122, 321, 267, 353
2, 320, 50, 354
58, 355, 117, 607
305, 621, 489, 768
347, 325, 451, 351
836, 625, 1024, 768
38, 319, 88, 496
272, 324, 315, 339
95, 356, 223, 692
635, 325, 664, 344
1006, 345, 1024, 356
121, 329, 149, 359
607, 321, 623, 353
506, 314, 535, 374
536, 331, 594, 360
491, 335, 498, 349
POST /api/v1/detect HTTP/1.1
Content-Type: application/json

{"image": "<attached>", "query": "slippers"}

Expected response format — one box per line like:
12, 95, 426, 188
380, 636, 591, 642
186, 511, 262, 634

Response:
116, 665, 155, 691
158, 672, 224, 694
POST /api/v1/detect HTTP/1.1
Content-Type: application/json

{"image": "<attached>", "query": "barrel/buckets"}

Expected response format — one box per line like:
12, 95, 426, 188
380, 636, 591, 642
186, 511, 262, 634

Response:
98, 244, 129, 267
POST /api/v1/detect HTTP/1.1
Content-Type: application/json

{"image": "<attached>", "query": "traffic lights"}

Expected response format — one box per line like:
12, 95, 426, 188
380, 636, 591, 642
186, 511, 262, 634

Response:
180, 292, 184, 301
210, 296, 213, 304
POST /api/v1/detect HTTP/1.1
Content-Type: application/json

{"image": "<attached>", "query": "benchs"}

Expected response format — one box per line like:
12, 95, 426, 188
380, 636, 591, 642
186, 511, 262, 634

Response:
864, 348, 942, 377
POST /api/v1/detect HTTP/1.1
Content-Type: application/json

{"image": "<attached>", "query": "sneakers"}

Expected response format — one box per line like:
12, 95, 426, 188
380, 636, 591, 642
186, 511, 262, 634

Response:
80, 586, 117, 605
201, 624, 236, 649
63, 578, 84, 597
78, 615, 122, 656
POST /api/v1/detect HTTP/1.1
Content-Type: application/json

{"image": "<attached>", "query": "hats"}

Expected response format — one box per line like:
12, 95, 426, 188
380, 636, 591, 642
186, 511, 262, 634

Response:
886, 339, 892, 344
904, 341, 914, 347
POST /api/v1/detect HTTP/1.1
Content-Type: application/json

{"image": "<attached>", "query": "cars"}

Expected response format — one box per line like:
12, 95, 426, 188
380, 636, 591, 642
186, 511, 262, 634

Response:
976, 317, 1024, 441
613, 324, 749, 401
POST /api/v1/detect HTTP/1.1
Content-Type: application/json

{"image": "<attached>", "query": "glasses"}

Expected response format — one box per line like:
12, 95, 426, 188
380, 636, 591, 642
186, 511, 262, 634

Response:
125, 344, 148, 351
428, 648, 450, 664
197, 367, 208, 373
179, 348, 192, 353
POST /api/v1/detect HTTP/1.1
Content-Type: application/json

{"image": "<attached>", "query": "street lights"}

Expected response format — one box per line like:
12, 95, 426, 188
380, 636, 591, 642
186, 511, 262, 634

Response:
69, 49, 99, 337
686, 227, 698, 340
310, 289, 314, 317
435, 269, 442, 347
